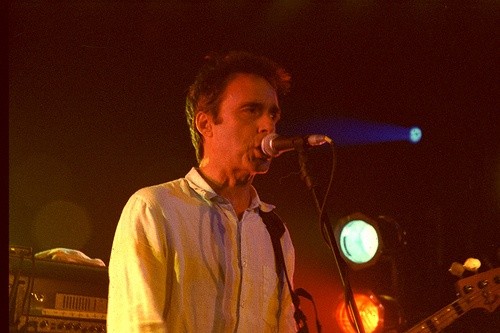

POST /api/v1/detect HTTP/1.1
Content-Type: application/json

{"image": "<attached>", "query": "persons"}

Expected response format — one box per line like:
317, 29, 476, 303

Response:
106, 50, 298, 333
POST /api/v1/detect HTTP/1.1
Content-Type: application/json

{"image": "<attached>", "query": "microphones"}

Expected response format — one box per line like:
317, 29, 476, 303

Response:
261, 133, 326, 156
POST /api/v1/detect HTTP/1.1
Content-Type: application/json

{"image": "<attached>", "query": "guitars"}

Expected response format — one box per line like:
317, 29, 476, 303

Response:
400, 256, 500, 333
